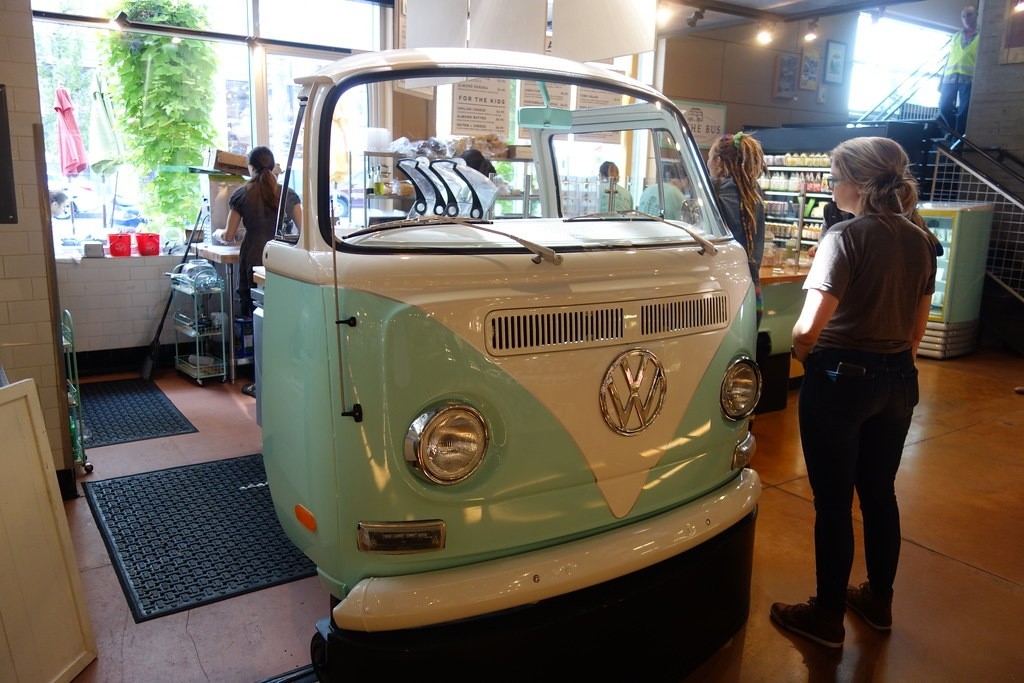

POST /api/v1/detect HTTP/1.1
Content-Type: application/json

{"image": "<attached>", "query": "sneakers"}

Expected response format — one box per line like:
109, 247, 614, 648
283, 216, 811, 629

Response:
771, 596, 846, 648
846, 581, 894, 629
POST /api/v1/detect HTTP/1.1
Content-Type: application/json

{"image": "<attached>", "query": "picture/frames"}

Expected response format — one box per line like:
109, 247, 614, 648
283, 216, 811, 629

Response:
823, 39, 847, 85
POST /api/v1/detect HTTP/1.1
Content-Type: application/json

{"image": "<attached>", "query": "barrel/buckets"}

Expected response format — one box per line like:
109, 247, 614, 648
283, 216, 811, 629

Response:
135, 232, 160, 256
108, 233, 132, 257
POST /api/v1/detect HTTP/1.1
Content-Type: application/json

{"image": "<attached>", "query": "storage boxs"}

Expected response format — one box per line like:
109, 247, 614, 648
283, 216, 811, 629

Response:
508, 145, 532, 158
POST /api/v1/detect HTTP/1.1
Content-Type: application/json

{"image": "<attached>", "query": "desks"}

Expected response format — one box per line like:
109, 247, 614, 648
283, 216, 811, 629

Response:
197, 245, 240, 386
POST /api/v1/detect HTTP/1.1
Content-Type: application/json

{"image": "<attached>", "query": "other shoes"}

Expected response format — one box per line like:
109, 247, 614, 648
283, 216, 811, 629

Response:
242, 383, 256, 398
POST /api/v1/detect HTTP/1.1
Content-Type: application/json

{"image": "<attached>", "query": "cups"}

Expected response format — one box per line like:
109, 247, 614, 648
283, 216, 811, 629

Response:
927, 228, 952, 305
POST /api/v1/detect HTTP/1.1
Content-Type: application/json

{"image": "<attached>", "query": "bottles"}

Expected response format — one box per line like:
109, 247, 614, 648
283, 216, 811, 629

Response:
180, 258, 218, 288
758, 152, 832, 240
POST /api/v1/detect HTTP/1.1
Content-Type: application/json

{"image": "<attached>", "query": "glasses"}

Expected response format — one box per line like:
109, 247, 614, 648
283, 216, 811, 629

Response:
827, 176, 853, 190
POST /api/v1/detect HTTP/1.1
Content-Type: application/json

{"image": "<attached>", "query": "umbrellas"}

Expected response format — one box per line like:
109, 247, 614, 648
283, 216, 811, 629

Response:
88, 67, 125, 228
54, 85, 86, 238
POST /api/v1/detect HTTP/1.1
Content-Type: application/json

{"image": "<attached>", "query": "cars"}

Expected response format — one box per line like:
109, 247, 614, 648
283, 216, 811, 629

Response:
47, 170, 193, 229
278, 170, 369, 218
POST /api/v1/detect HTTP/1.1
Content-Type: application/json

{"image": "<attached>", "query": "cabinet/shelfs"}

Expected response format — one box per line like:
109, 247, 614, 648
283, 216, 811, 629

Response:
170, 264, 228, 387
61, 309, 93, 473
764, 167, 832, 267
364, 151, 533, 228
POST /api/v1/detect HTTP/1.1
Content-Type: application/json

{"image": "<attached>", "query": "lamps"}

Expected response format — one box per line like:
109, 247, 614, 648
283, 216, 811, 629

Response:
756, 19, 776, 44
805, 16, 819, 40
687, 8, 705, 26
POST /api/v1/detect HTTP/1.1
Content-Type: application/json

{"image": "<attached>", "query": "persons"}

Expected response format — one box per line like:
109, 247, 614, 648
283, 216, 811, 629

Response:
770, 137, 936, 648
214, 146, 302, 398
808, 202, 856, 257
707, 132, 769, 331
599, 161, 634, 212
937, 4, 980, 153
640, 163, 689, 221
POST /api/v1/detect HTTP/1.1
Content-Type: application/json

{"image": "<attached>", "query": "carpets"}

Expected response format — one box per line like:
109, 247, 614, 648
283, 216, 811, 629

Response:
80, 453, 318, 624
67, 377, 200, 449
